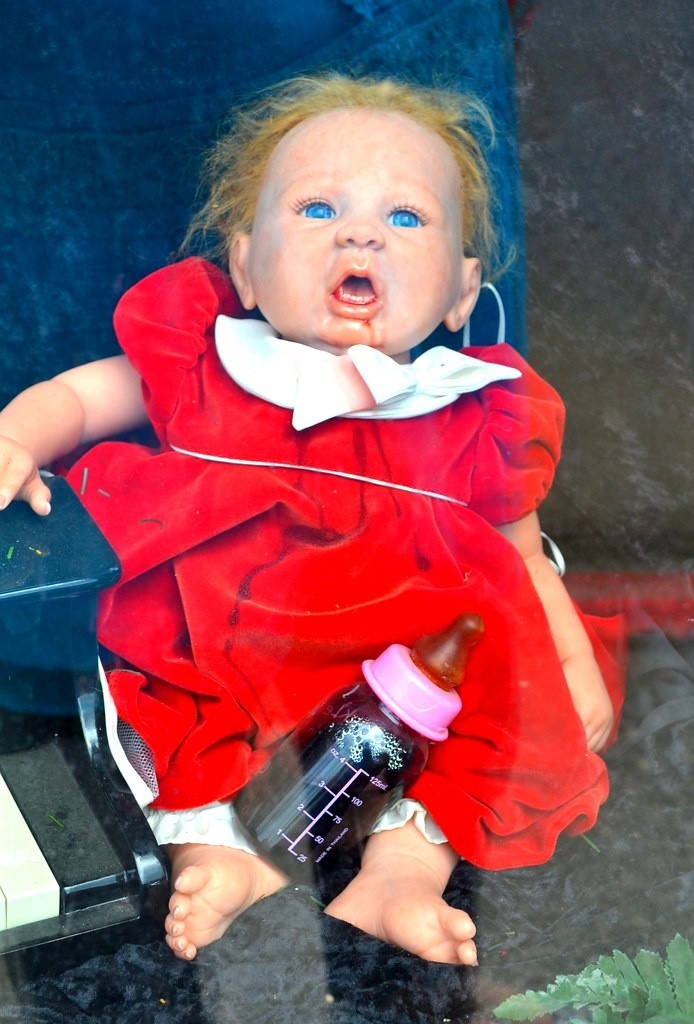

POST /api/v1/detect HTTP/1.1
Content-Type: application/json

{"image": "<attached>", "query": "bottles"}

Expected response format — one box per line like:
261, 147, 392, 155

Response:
230, 611, 486, 886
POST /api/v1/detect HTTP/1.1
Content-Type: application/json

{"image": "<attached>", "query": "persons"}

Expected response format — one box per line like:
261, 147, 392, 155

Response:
0, 76, 622, 966
